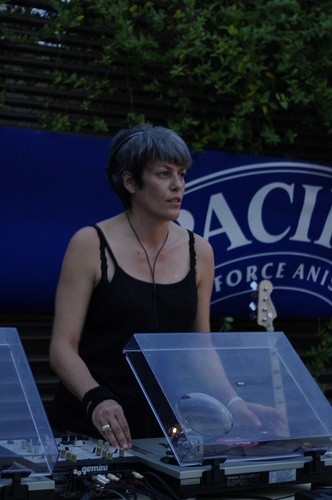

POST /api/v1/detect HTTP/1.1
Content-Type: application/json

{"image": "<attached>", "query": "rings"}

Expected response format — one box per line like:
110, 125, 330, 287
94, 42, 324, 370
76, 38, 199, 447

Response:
101, 424, 111, 434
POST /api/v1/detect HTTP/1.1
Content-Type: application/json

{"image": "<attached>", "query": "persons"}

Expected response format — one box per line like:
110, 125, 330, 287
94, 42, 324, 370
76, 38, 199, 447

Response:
50, 126, 287, 453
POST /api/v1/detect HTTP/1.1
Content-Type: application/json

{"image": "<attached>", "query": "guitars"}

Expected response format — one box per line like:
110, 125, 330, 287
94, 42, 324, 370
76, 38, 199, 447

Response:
249, 279, 300, 438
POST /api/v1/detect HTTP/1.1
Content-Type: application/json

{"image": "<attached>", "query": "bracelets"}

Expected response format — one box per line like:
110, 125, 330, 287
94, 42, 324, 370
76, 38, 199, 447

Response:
227, 397, 243, 409
